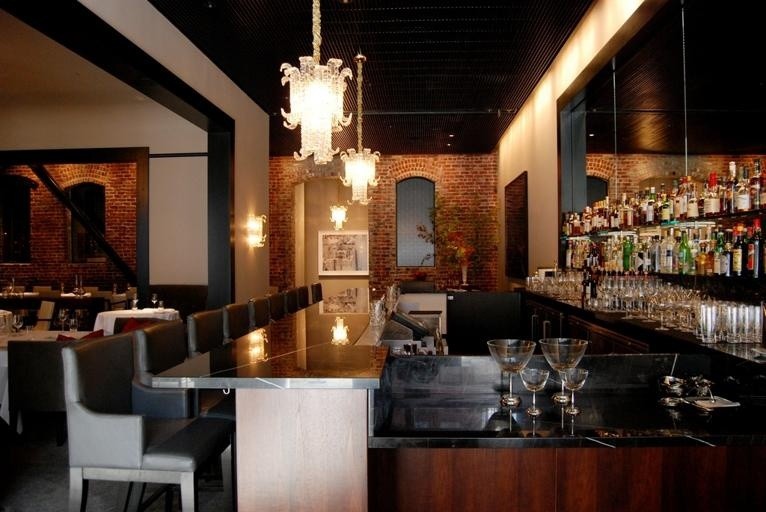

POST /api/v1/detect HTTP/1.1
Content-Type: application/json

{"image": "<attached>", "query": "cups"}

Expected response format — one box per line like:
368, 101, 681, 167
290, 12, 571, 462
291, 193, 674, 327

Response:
530, 268, 764, 343
367, 271, 400, 324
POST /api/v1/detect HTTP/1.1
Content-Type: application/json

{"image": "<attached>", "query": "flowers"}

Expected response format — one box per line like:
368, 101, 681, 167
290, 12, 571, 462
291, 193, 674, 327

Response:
417, 191, 502, 285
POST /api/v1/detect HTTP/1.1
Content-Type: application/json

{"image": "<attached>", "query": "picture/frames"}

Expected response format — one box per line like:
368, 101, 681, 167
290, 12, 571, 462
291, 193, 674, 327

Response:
318, 286, 370, 316
318, 230, 370, 277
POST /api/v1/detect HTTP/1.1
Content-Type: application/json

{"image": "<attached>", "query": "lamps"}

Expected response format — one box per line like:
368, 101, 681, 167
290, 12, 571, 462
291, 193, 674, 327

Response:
247, 214, 267, 248
329, 317, 352, 360
325, 203, 349, 229
338, 53, 382, 206
280, 1, 353, 166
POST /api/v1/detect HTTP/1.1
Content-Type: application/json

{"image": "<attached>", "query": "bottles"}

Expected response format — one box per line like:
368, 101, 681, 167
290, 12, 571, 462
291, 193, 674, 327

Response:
565, 221, 763, 276
562, 158, 766, 237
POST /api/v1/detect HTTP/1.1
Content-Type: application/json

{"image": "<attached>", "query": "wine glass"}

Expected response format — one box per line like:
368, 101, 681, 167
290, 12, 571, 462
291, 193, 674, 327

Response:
486, 335, 588, 416
132, 293, 164, 311
12, 308, 79, 339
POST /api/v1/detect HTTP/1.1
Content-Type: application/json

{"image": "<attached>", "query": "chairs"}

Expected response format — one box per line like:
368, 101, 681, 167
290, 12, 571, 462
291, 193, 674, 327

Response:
400, 280, 434, 292
447, 291, 523, 354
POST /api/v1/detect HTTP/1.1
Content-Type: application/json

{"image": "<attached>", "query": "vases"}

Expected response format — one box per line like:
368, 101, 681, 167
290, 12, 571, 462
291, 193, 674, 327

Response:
461, 265, 468, 285
